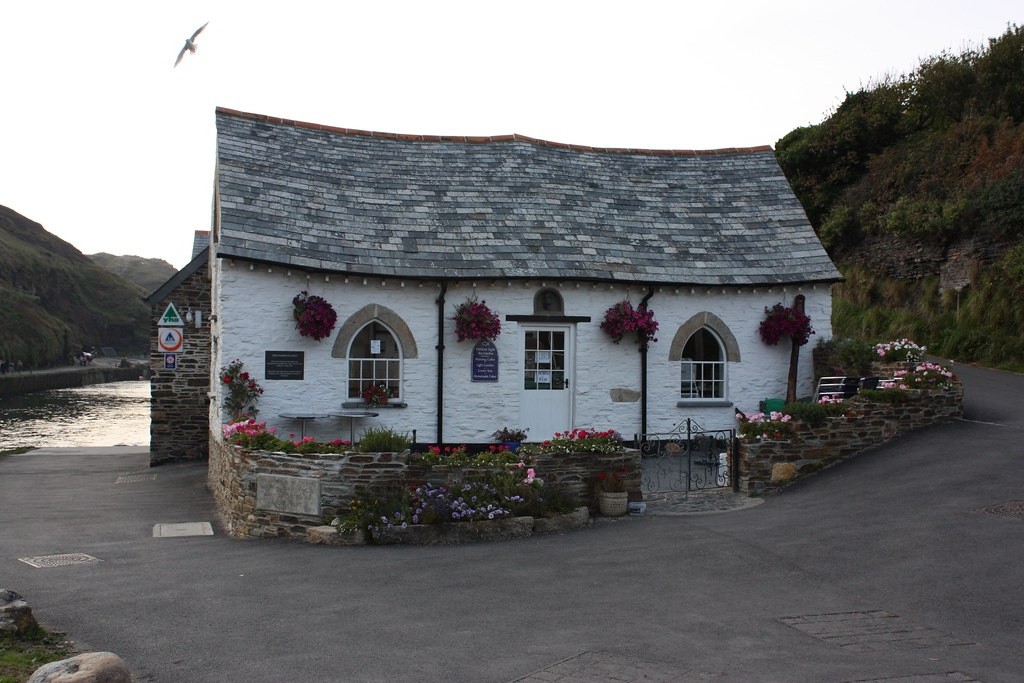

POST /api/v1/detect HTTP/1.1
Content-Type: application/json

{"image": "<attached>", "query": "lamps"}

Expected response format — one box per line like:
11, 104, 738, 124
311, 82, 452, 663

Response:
185, 307, 193, 322
544, 293, 552, 310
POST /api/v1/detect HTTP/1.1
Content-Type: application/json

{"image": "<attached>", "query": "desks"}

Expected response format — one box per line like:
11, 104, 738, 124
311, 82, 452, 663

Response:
329, 412, 379, 449
278, 413, 328, 444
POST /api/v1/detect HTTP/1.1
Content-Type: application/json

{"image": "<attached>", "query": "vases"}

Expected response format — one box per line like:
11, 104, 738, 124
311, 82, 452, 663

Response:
502, 441, 520, 454
598, 491, 628, 515
377, 395, 389, 404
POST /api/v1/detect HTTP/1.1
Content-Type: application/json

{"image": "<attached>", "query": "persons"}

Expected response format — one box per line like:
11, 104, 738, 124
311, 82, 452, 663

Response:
80, 355, 87, 367
0, 359, 23, 374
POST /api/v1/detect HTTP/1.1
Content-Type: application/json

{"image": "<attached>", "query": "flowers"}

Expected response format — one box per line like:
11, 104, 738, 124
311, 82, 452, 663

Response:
515, 427, 624, 456
492, 427, 529, 442
219, 358, 353, 454
735, 411, 792, 441
474, 445, 512, 466
588, 460, 641, 493
505, 460, 552, 519
363, 385, 390, 408
818, 394, 844, 417
422, 443, 469, 467
881, 360, 956, 390
452, 297, 501, 343
760, 303, 816, 346
601, 301, 659, 352
872, 338, 928, 362
339, 478, 523, 537
291, 291, 337, 341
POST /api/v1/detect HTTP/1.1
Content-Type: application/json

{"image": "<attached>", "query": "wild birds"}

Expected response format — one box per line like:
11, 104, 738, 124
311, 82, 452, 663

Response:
172, 19, 211, 70
